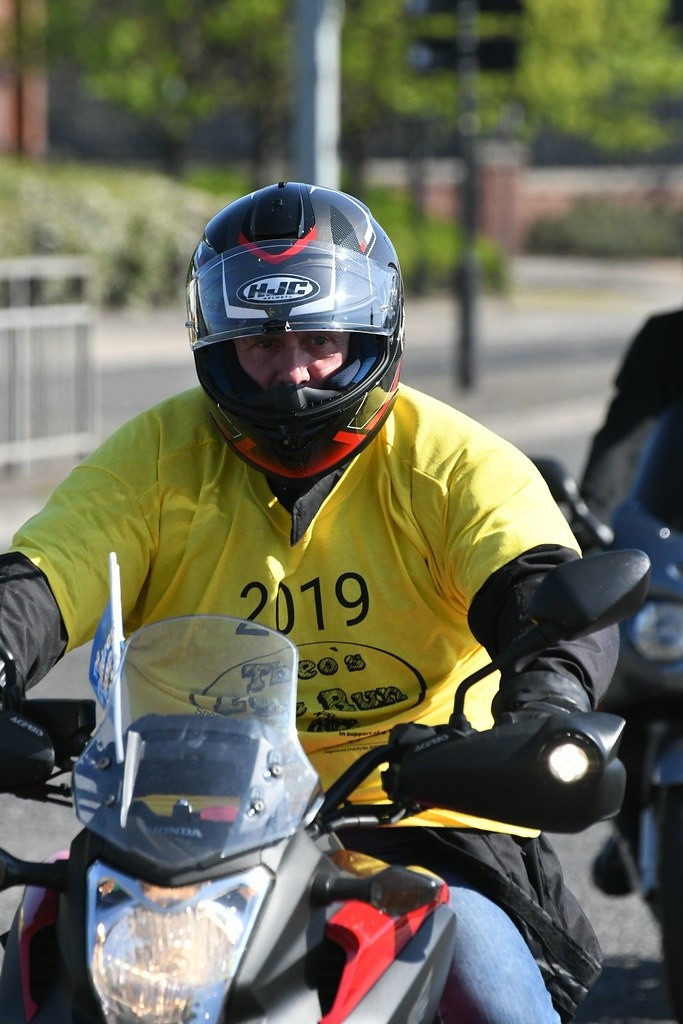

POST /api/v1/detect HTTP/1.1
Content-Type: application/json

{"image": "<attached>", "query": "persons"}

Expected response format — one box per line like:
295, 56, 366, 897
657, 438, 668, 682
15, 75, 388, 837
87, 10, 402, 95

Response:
0, 186, 622, 1024
586, 311, 682, 892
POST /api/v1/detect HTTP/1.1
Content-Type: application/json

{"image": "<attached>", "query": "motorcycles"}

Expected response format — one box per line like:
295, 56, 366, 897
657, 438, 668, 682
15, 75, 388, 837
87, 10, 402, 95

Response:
1, 546, 658, 1024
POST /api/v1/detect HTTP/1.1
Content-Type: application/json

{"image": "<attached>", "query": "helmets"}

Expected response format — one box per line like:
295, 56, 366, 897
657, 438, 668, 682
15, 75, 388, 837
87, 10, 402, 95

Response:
187, 181, 405, 485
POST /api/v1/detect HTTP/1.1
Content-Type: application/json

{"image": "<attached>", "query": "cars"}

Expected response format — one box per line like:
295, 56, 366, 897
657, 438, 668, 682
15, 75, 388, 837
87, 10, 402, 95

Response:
530, 297, 682, 1019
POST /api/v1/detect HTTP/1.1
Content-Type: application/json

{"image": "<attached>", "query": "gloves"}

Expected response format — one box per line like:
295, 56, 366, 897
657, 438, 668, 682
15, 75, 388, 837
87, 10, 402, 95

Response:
494, 672, 591, 732
